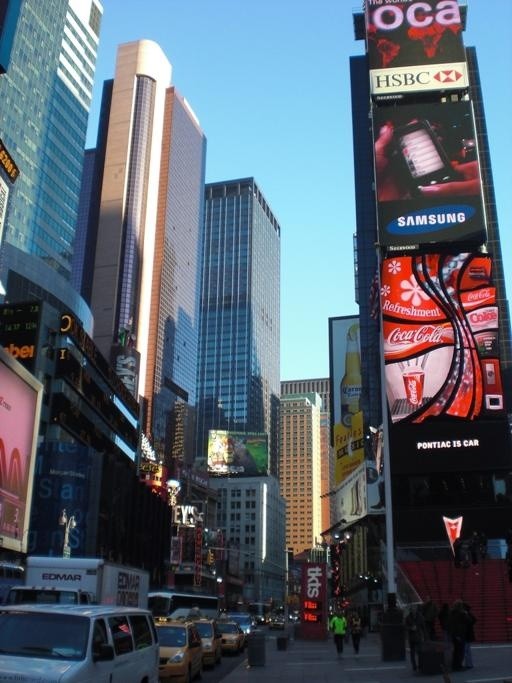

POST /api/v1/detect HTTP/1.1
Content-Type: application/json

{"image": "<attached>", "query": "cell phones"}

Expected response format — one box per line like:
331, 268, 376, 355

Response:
394, 120, 462, 196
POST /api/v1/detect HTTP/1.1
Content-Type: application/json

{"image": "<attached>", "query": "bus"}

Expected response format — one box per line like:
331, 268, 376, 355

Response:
250, 603, 272, 625
147, 592, 220, 621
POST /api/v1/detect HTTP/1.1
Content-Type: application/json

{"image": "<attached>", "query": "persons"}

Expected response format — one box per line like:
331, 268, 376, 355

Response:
374, 115, 480, 204
332, 610, 347, 655
343, 614, 352, 644
348, 612, 365, 654
403, 598, 478, 674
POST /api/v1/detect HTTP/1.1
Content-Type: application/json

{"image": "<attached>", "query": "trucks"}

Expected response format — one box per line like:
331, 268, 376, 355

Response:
8, 555, 150, 611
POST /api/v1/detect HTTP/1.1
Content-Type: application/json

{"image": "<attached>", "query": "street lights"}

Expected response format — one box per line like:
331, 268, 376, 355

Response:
60, 509, 76, 561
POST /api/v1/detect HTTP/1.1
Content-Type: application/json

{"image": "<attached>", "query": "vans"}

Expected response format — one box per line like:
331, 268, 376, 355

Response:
0, 606, 160, 682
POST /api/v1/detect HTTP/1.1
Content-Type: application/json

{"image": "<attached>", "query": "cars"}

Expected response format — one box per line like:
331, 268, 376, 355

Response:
154, 613, 257, 682
270, 614, 298, 630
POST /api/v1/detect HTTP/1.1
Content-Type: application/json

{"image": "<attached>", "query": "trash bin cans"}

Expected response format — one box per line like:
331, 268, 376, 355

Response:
379, 624, 406, 661
248, 633, 266, 666
